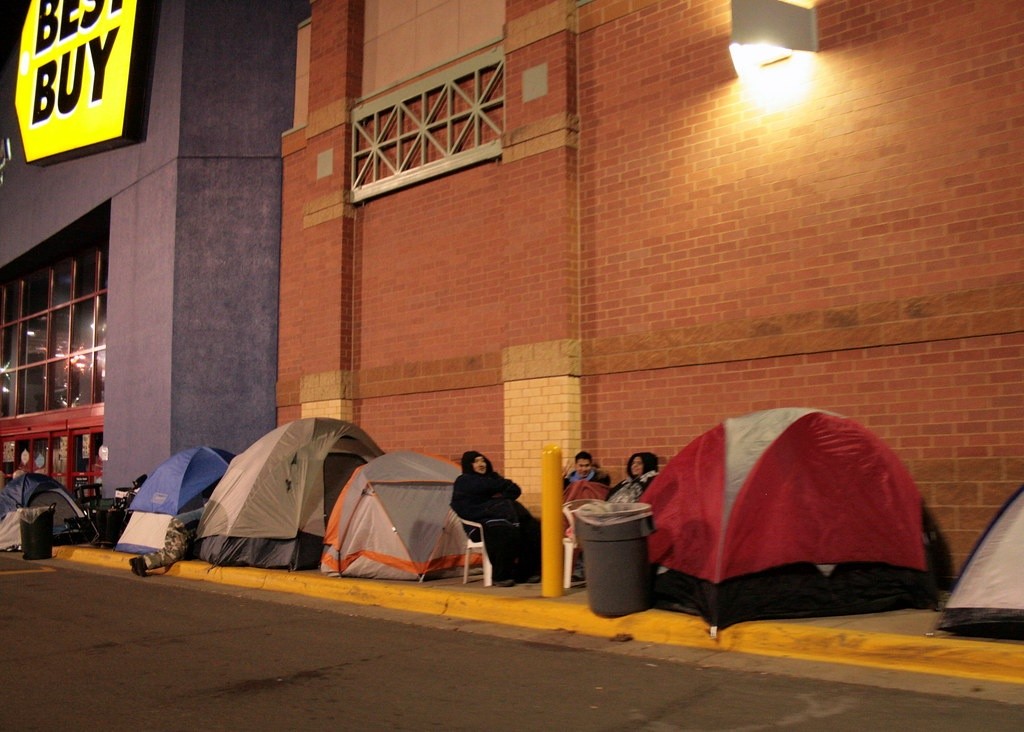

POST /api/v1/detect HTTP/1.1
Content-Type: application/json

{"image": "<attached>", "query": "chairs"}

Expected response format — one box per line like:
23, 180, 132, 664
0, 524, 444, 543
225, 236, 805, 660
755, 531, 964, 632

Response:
459, 517, 493, 589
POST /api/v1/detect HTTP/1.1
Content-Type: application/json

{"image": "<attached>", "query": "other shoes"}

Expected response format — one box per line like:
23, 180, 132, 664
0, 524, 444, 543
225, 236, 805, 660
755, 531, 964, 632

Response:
516, 576, 541, 582
129, 557, 148, 577
494, 580, 514, 587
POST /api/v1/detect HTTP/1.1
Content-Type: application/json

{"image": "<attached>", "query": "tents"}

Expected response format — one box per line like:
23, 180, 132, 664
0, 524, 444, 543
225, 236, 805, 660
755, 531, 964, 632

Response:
191, 415, 387, 571
635, 406, 952, 634
116, 447, 234, 554
320, 451, 486, 587
933, 483, 1024, 642
0, 473, 94, 552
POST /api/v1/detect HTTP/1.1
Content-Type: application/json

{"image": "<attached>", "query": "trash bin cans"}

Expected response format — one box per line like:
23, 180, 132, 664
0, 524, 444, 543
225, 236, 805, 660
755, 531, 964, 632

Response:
16, 502, 56, 560
571, 503, 658, 617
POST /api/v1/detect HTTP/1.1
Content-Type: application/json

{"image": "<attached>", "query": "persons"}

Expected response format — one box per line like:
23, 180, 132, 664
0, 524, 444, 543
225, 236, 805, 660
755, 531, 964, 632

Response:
562, 453, 611, 489
571, 451, 659, 586
127, 506, 205, 576
451, 450, 541, 585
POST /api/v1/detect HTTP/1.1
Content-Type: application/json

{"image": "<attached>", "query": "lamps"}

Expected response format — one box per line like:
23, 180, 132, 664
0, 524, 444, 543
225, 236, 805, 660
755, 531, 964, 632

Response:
729, 1, 819, 84
